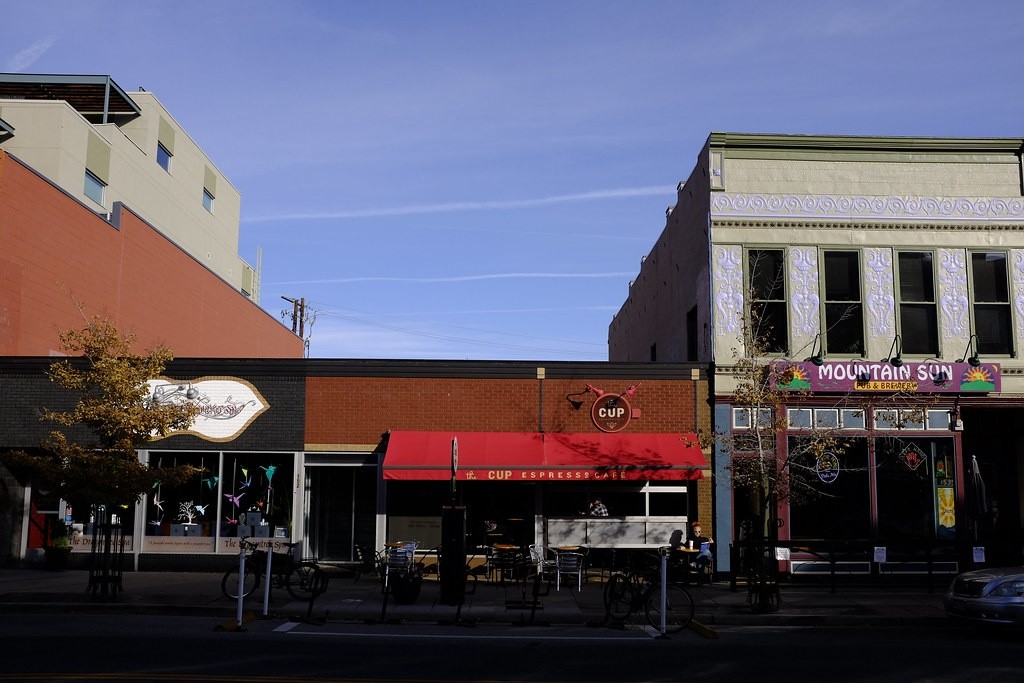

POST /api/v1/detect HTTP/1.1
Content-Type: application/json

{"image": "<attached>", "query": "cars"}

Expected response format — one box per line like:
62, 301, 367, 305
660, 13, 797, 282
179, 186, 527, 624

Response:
944, 568, 1024, 624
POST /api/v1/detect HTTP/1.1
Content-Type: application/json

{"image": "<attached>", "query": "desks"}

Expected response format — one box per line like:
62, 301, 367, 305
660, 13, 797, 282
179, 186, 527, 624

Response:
555, 546, 580, 552
384, 543, 405, 587
676, 548, 699, 587
489, 544, 521, 589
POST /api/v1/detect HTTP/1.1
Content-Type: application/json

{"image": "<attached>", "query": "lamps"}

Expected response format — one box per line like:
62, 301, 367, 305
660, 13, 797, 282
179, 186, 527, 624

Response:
625, 382, 642, 398
881, 335, 904, 367
566, 383, 603, 399
805, 334, 823, 366
957, 335, 981, 366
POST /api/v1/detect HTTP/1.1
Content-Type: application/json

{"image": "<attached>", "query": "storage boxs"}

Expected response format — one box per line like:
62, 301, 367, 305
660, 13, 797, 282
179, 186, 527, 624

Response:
170, 524, 203, 536
275, 526, 288, 538
238, 512, 269, 537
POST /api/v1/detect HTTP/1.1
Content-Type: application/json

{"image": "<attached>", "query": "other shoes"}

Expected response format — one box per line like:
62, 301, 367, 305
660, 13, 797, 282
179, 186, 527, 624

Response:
698, 579, 706, 592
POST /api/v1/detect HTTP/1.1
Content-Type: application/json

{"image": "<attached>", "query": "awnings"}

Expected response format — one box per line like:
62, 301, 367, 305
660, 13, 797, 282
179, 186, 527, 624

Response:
381, 431, 709, 481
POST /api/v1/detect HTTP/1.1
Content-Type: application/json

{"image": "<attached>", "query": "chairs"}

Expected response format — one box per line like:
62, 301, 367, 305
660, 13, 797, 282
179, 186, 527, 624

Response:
353, 545, 383, 584
528, 544, 590, 592
384, 542, 419, 587
687, 542, 715, 586
492, 548, 521, 588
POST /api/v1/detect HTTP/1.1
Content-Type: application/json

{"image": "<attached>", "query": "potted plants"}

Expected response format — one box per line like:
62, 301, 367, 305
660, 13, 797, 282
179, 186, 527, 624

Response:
44, 518, 81, 573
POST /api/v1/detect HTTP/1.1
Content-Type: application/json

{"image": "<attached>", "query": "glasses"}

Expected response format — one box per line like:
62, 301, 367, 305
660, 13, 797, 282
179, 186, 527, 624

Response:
694, 530, 700, 533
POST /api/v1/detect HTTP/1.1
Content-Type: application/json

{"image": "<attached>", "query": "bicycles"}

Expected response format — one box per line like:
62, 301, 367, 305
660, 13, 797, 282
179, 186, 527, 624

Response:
601, 551, 693, 636
222, 535, 328, 601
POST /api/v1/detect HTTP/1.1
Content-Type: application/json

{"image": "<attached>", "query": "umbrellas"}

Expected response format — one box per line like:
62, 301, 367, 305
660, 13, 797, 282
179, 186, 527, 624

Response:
961, 454, 985, 541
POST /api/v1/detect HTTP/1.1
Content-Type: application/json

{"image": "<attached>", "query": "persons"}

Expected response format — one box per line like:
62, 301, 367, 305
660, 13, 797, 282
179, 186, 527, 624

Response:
584, 496, 609, 517
684, 522, 710, 587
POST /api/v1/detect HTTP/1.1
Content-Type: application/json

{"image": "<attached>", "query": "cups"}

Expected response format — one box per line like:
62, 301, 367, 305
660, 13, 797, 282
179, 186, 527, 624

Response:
689, 540, 693, 550
